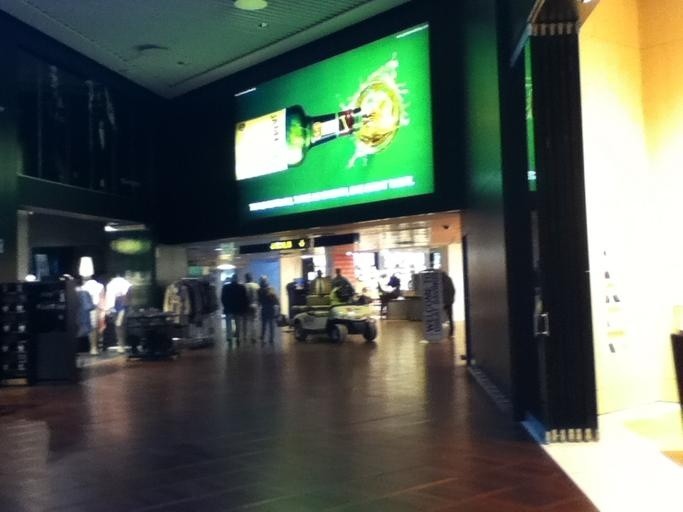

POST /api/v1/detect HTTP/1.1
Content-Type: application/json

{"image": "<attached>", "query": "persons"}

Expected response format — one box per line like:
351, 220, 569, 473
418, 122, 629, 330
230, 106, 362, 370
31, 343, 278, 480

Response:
440, 272, 455, 336
388, 273, 398, 286
74, 265, 132, 355
308, 268, 354, 302
221, 272, 279, 344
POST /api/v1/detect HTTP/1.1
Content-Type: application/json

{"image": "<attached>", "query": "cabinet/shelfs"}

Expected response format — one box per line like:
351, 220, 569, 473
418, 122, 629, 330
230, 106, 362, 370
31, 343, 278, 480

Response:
125, 311, 181, 360
0, 282, 66, 386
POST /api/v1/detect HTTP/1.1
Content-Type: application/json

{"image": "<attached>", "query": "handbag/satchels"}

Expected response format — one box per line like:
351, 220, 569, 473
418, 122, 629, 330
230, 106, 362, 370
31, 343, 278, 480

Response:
273, 305, 279, 318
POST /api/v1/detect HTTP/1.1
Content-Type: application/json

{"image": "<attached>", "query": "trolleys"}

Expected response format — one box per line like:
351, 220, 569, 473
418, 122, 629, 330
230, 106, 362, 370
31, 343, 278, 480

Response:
123, 314, 189, 360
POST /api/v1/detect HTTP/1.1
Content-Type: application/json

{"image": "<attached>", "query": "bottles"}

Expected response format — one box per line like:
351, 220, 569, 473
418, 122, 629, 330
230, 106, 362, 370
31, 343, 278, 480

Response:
233, 105, 362, 183
